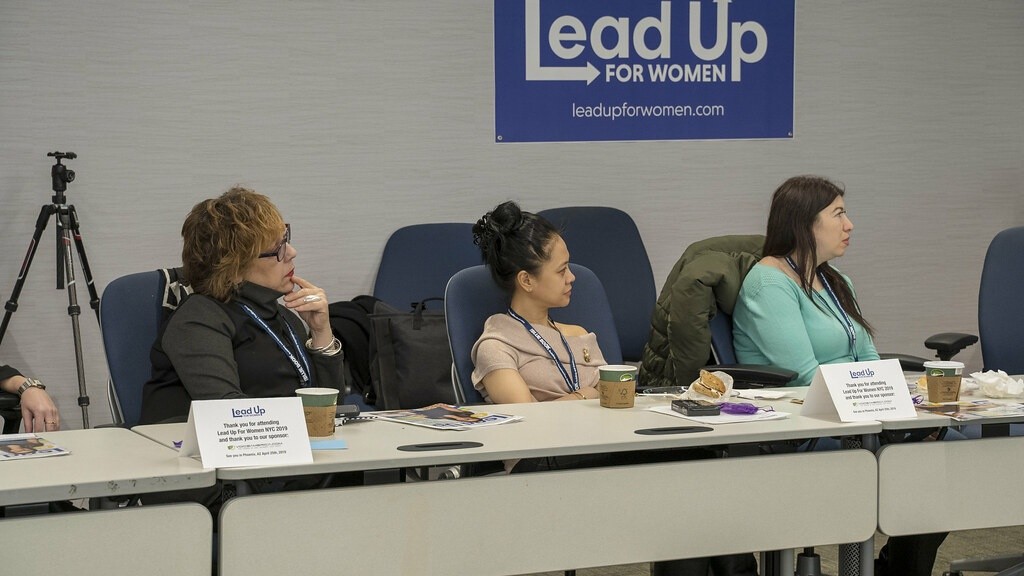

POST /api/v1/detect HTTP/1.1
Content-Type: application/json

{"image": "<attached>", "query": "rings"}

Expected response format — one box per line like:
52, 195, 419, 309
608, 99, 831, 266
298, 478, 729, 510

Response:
46, 420, 57, 425
305, 295, 320, 303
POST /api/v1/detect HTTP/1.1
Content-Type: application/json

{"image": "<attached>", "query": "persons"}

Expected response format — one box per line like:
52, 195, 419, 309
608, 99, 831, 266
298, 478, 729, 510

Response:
471, 200, 759, 576
732, 174, 949, 576
0, 361, 62, 436
139, 183, 364, 537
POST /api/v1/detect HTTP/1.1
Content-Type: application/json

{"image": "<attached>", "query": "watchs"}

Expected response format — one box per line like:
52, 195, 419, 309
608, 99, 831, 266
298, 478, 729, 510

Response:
17, 377, 45, 397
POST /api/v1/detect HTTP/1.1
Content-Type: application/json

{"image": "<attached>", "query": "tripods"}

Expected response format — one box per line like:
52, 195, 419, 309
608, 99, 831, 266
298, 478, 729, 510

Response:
0, 152, 101, 430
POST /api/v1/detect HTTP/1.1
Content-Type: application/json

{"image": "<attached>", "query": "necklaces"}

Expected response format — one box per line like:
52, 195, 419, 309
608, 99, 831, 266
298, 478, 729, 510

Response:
786, 255, 860, 362
507, 306, 580, 393
238, 302, 312, 387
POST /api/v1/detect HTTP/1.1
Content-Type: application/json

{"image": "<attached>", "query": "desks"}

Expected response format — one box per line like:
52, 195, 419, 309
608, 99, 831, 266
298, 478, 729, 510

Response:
0, 369, 1024, 576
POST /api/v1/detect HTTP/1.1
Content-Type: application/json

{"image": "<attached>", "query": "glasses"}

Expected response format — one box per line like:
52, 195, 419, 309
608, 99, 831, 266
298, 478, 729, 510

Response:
252, 223, 291, 262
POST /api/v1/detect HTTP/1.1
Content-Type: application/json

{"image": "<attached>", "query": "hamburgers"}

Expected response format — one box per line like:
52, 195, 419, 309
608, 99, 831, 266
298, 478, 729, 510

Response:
692, 369, 725, 399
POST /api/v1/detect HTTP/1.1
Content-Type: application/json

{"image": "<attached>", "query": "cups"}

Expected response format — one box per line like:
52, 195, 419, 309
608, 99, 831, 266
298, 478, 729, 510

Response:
294, 387, 339, 438
923, 360, 965, 403
598, 364, 638, 409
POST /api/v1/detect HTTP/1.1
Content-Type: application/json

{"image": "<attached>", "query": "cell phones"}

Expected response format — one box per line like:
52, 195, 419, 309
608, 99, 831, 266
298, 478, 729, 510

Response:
335, 404, 360, 417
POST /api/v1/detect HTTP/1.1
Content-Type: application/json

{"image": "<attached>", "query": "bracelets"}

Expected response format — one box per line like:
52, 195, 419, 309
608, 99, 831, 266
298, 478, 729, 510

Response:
574, 390, 588, 400
304, 332, 335, 354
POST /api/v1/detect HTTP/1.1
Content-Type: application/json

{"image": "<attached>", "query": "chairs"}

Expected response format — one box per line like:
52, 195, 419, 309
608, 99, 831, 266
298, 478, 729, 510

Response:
372, 204, 971, 576
98, 267, 251, 504
923, 228, 1024, 439
